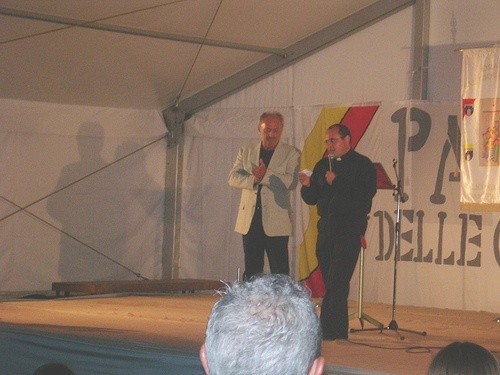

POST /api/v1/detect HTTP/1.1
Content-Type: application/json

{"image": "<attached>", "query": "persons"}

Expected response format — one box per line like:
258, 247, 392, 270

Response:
298, 124, 377, 341
427, 341, 500, 375
228, 112, 302, 282
200, 274, 325, 375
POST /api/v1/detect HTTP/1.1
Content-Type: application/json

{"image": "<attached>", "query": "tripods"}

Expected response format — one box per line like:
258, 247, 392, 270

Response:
347, 158, 427, 341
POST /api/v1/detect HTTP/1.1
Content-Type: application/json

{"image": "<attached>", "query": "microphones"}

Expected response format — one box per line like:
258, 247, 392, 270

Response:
328, 152, 334, 186
